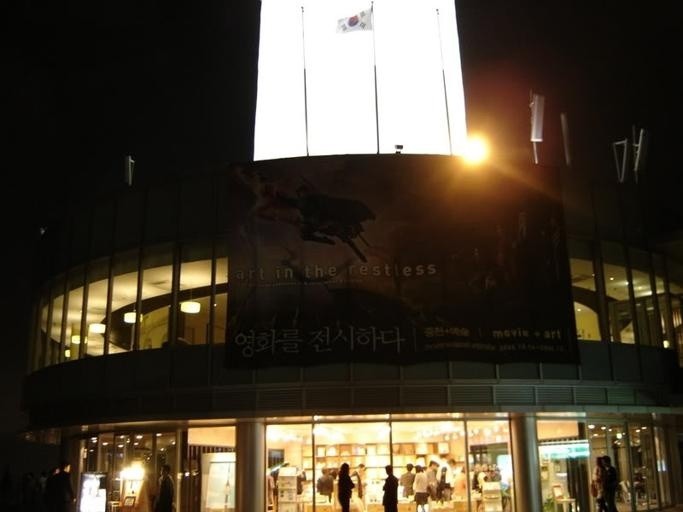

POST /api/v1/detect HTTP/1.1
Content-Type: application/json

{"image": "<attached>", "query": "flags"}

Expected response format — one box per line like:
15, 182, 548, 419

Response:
335, 8, 372, 32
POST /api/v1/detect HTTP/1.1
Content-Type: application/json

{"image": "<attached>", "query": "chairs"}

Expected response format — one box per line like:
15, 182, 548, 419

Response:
551, 483, 576, 511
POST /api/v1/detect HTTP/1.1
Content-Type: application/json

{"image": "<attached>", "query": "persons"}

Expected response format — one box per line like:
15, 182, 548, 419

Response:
267, 462, 307, 512
157, 464, 174, 512
383, 456, 502, 512
595, 456, 620, 512
44, 462, 77, 512
316, 463, 365, 512
